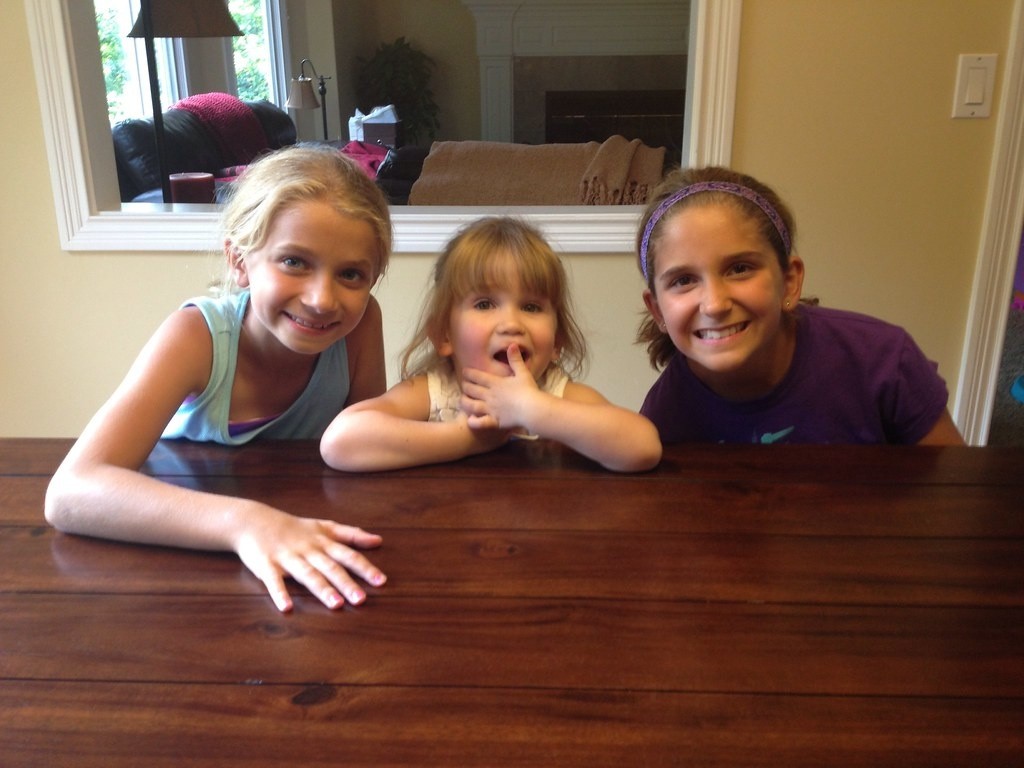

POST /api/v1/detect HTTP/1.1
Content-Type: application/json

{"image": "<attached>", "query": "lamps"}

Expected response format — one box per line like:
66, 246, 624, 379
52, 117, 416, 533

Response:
284, 58, 330, 144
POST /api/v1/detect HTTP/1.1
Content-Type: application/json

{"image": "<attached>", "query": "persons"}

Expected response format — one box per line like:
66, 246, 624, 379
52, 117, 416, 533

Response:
43, 140, 397, 617
319, 216, 663, 477
632, 164, 969, 446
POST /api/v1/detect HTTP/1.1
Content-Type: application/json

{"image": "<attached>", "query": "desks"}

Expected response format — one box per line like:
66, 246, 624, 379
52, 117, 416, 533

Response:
0, 431, 1024, 765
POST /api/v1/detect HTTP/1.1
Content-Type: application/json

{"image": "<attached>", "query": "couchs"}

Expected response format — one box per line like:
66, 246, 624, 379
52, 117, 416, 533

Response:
111, 92, 298, 208
371, 138, 673, 206
